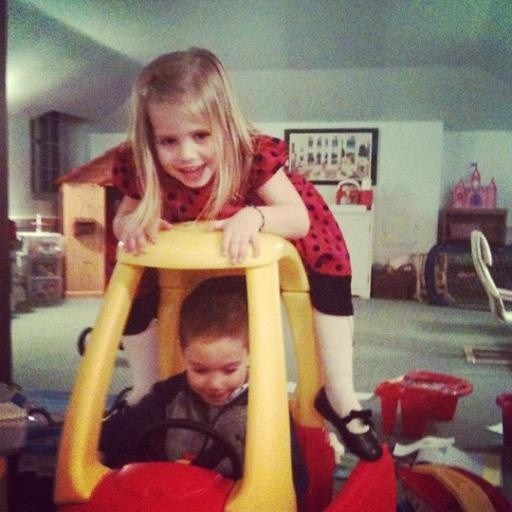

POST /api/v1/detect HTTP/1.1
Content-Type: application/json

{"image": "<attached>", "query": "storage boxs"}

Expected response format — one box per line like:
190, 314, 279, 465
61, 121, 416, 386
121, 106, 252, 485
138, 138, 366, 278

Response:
16, 231, 65, 301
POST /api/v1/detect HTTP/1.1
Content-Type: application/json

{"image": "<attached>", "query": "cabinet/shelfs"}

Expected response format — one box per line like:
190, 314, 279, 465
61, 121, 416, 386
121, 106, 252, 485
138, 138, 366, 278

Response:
438, 208, 506, 299
59, 182, 106, 296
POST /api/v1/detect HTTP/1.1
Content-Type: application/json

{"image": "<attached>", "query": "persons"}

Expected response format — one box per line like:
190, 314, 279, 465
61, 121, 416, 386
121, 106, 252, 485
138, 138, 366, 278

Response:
99, 279, 309, 502
114, 46, 383, 464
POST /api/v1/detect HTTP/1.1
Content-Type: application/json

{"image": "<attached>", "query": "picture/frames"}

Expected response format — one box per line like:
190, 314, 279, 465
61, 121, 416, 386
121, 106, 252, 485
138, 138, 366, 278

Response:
284, 128, 378, 185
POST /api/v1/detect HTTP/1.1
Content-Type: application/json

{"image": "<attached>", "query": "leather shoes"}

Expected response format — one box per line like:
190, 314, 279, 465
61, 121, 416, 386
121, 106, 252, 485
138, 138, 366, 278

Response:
314, 386, 381, 461
102, 396, 163, 431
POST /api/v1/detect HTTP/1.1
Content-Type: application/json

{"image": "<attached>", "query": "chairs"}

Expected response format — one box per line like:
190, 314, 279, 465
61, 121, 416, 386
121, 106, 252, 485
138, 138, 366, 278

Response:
465, 231, 512, 365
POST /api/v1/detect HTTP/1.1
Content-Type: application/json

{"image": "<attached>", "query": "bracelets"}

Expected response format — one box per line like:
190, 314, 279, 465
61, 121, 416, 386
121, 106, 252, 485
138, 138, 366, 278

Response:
244, 203, 266, 234
